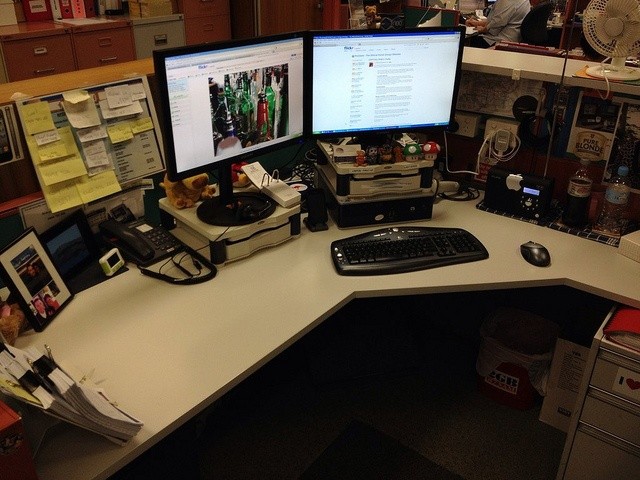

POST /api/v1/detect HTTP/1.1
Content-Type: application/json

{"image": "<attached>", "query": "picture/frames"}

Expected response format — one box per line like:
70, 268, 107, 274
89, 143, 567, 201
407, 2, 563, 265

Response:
41, 209, 108, 295
0, 226, 74, 333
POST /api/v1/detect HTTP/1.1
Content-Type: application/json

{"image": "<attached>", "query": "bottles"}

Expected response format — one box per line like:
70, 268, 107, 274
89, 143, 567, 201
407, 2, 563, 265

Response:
562, 157, 593, 227
594, 166, 630, 234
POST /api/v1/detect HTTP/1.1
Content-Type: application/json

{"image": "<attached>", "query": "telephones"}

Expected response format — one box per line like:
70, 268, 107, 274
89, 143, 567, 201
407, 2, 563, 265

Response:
97, 217, 184, 270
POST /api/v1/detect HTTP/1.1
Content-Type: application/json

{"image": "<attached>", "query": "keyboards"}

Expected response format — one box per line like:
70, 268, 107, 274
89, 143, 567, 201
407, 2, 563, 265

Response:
330, 227, 489, 277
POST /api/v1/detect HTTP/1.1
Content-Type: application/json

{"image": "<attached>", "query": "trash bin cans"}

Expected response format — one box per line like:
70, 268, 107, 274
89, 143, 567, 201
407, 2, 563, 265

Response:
477, 309, 559, 410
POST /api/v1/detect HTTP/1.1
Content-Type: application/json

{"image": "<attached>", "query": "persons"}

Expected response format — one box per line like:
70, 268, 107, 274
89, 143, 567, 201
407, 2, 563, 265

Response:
32, 297, 54, 325
465, 0, 531, 48
43, 294, 60, 312
26, 264, 52, 296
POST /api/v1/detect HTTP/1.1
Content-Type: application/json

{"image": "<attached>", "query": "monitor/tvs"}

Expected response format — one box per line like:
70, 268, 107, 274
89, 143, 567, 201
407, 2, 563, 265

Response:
152, 31, 310, 227
421, 0, 489, 24
307, 26, 465, 151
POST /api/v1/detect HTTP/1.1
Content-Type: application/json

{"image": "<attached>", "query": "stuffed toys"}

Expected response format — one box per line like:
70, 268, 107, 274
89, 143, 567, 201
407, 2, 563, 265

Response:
231, 162, 252, 188
365, 5, 381, 29
159, 171, 217, 210
353, 144, 403, 169
0, 297, 29, 346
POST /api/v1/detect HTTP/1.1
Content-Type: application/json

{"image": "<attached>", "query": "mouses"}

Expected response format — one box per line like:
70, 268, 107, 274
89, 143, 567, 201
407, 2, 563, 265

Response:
521, 241, 549, 266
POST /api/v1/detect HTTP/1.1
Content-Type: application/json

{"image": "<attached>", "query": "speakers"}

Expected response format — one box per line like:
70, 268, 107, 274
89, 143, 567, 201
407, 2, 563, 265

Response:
303, 187, 328, 232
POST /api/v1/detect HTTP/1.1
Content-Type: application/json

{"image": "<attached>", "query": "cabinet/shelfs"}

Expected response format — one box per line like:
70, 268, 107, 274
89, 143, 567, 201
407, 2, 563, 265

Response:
234, 1, 322, 40
179, 1, 234, 47
132, 13, 187, 61
558, 0, 610, 62
50, 13, 136, 72
552, 304, 639, 480
0, 21, 78, 84
323, 0, 424, 30
0, 43, 10, 84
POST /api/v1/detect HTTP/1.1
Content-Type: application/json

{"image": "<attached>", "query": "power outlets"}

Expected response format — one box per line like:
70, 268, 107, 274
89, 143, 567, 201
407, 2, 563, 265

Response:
448, 112, 482, 138
483, 117, 521, 146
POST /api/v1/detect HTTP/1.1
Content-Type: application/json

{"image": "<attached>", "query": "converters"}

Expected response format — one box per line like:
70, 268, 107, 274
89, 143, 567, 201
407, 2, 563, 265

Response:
493, 130, 510, 154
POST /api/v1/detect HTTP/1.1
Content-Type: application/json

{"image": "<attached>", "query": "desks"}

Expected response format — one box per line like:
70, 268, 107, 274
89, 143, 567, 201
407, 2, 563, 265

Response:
0, 188, 639, 478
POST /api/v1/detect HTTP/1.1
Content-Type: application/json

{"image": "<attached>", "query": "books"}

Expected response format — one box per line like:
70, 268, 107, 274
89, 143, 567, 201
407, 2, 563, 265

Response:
1, 347, 144, 447
240, 161, 302, 209
